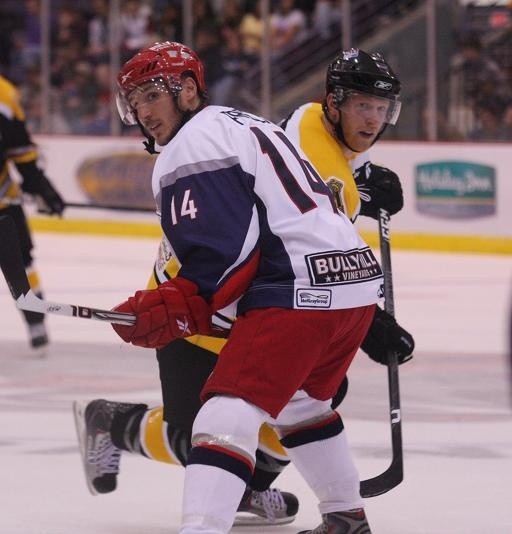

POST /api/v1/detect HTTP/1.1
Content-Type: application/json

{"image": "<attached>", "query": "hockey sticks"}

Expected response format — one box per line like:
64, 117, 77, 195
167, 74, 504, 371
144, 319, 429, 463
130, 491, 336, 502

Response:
2, 212, 138, 325
360, 168, 402, 497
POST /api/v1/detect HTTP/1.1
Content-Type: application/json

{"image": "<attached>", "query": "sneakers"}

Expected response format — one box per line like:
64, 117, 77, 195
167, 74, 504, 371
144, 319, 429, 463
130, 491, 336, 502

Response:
85, 399, 148, 493
237, 487, 299, 519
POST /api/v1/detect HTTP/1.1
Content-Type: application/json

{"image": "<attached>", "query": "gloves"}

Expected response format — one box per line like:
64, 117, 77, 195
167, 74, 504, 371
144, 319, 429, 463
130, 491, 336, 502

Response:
110, 278, 214, 348
352, 160, 404, 220
358, 306, 415, 365
14, 158, 65, 215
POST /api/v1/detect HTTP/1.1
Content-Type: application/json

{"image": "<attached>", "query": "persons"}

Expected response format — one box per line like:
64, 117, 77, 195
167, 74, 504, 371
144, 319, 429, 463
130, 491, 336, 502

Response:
85, 48, 414, 519
0, 77, 64, 352
429, 0, 512, 141
110, 40, 385, 534
0, 0, 342, 134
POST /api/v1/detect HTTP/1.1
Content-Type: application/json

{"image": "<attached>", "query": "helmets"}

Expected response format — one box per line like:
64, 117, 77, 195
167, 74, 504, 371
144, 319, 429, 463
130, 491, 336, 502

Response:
115, 42, 208, 96
327, 48, 401, 99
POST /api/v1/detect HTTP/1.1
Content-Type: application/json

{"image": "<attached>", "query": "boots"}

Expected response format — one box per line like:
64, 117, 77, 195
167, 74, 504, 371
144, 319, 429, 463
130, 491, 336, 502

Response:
26, 325, 48, 346
297, 508, 372, 534
